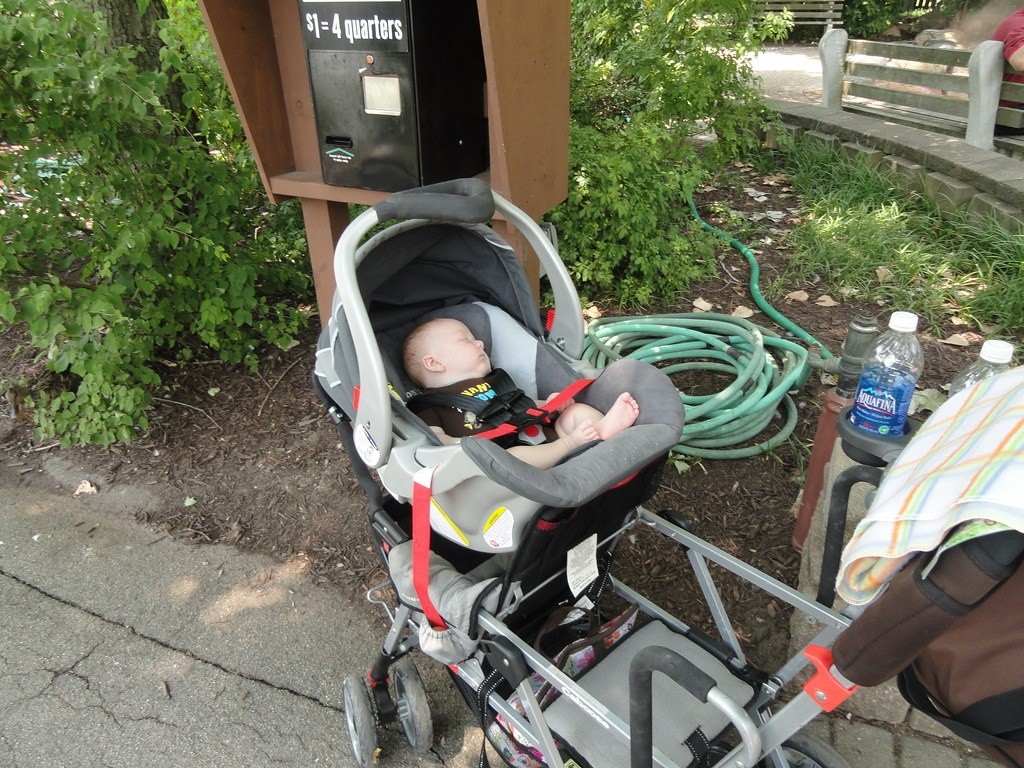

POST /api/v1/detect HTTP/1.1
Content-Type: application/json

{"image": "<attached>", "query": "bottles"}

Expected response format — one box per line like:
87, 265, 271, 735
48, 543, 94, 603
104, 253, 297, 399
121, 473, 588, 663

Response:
850, 311, 924, 436
948, 339, 1014, 400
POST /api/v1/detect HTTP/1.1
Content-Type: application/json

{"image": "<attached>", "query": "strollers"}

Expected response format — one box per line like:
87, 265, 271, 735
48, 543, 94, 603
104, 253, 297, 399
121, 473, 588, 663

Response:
309, 178, 1023, 768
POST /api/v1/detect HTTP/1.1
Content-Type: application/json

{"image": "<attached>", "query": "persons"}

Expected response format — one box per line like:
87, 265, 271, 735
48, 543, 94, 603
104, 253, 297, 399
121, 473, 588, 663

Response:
990, 7, 1024, 138
403, 318, 640, 471
